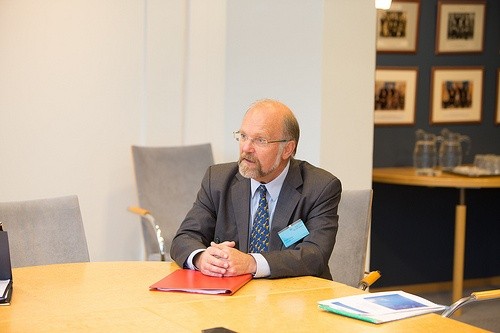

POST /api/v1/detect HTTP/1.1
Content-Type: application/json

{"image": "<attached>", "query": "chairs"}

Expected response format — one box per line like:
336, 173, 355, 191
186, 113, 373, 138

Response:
127, 143, 217, 261
0, 195, 91, 268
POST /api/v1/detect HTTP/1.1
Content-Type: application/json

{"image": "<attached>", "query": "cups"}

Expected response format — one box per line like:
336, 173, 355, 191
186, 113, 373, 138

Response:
474, 154, 500, 176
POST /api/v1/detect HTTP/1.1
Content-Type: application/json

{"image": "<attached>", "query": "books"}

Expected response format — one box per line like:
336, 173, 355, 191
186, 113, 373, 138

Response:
318, 290, 449, 324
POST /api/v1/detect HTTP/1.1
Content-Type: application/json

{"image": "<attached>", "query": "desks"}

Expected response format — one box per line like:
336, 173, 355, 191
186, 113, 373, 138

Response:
374, 167, 500, 315
0, 261, 493, 333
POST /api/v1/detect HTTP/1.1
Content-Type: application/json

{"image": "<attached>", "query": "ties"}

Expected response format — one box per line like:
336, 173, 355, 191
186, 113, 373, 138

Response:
248, 185, 270, 253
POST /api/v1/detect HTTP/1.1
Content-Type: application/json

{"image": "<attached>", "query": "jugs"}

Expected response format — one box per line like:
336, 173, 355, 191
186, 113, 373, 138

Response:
438, 129, 471, 168
413, 129, 442, 177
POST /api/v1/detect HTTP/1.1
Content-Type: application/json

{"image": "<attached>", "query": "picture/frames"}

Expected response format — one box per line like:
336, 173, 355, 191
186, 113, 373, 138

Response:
373, 66, 419, 125
435, 1, 486, 56
429, 66, 484, 126
376, 0, 420, 54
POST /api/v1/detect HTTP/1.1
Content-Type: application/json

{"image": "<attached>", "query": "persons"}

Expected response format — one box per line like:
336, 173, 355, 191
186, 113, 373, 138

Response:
170, 99, 342, 281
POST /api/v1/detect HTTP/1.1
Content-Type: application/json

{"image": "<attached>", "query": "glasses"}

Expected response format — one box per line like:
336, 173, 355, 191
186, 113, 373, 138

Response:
232, 130, 290, 150
